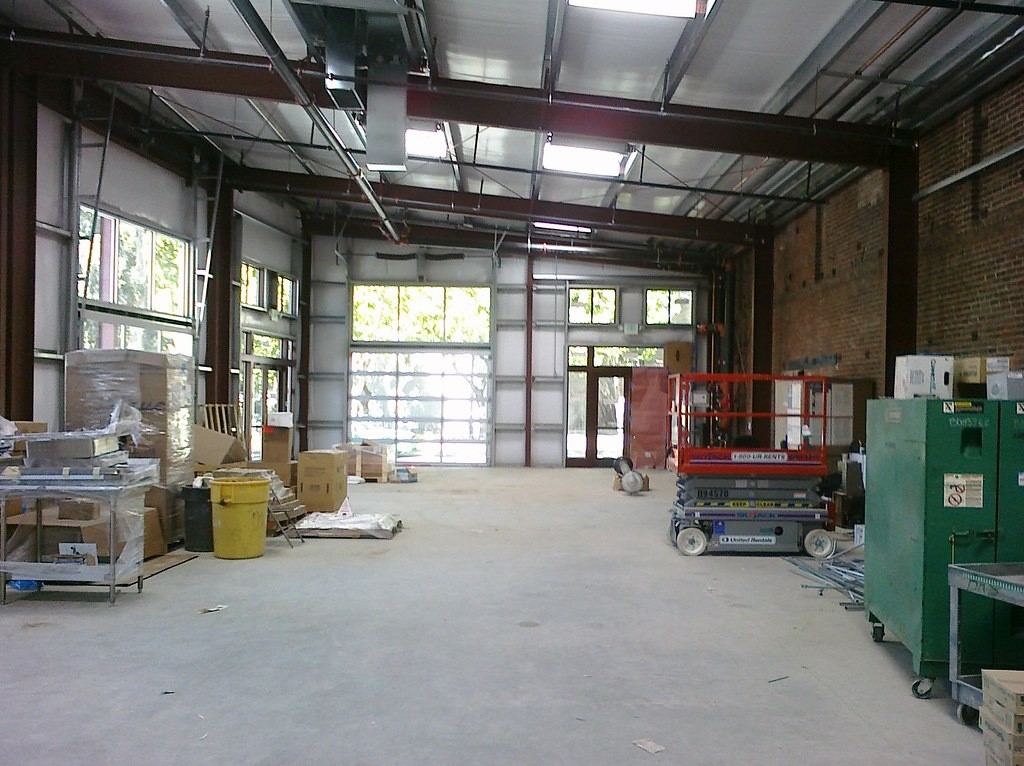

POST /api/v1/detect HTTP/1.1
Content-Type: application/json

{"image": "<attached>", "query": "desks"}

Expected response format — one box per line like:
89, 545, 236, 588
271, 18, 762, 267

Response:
0, 484, 152, 606
948, 562, 1024, 725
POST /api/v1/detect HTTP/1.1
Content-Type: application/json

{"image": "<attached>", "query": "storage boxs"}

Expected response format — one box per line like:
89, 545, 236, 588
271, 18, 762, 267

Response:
13, 421, 48, 436
27, 433, 118, 458
297, 449, 349, 512
388, 467, 420, 483
228, 460, 298, 485
262, 425, 294, 461
192, 424, 246, 466
267, 411, 293, 427
57, 498, 101, 521
977, 668, 1024, 766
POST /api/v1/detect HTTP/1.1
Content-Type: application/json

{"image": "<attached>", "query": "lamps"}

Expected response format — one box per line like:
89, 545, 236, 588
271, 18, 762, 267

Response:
531, 221, 592, 234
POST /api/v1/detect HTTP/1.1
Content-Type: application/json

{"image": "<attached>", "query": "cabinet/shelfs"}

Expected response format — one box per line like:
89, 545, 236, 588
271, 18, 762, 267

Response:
64, 349, 196, 554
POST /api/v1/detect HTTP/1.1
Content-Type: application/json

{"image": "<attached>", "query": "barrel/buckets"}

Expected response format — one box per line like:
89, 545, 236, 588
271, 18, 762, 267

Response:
180, 476, 272, 560
